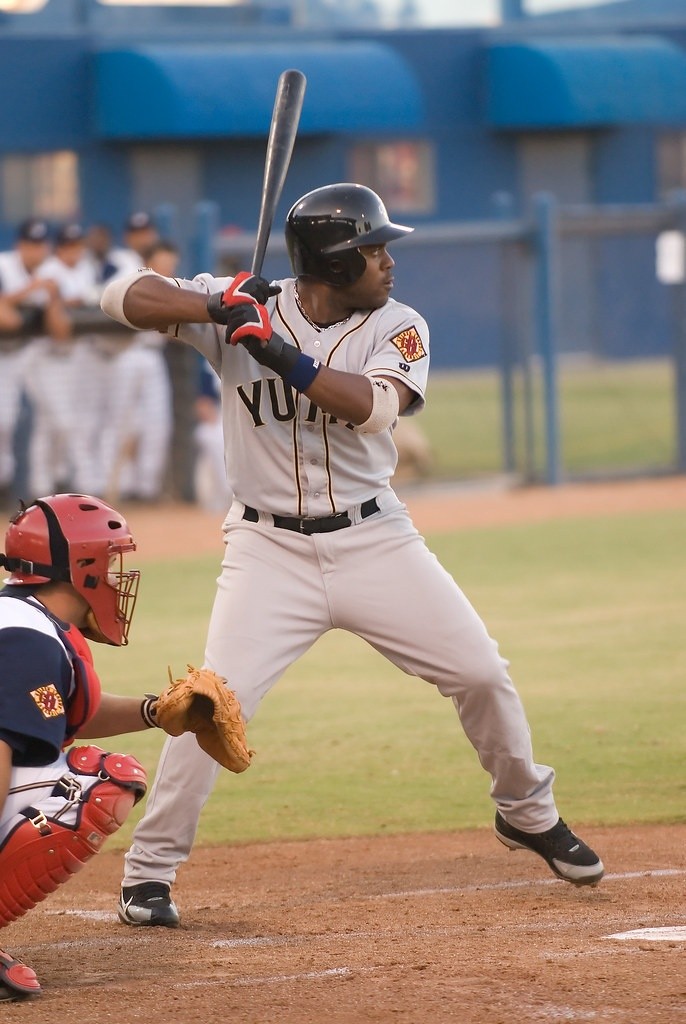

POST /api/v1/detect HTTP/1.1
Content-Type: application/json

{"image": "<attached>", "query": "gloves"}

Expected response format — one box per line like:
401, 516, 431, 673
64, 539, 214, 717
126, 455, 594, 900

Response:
224, 303, 285, 365
207, 271, 282, 325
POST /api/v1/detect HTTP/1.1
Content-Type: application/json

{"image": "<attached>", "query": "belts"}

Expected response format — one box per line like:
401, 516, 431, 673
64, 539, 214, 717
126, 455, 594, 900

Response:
243, 497, 381, 536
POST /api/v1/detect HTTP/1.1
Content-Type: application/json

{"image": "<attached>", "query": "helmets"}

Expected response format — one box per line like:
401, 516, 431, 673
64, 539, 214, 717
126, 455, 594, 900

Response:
2, 492, 139, 646
124, 210, 156, 232
16, 216, 50, 242
285, 182, 416, 289
56, 222, 90, 245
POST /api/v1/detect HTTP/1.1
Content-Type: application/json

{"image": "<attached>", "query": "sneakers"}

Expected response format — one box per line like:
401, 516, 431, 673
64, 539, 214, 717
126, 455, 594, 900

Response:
117, 882, 180, 929
493, 809, 605, 886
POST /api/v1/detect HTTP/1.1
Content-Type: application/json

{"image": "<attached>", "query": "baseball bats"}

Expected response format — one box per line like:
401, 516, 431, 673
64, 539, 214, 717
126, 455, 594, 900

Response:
249, 71, 306, 276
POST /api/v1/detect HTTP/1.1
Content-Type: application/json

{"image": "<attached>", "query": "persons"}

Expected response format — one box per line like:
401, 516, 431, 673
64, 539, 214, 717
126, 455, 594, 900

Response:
99, 183, 605, 922
0, 494, 252, 1002
0, 213, 234, 509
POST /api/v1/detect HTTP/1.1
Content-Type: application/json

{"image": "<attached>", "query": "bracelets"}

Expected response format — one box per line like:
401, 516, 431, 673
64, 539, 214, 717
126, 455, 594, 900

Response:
140, 692, 159, 728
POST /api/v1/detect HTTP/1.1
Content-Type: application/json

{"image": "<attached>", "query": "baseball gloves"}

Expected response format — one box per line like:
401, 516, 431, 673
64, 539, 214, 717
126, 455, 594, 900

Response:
144, 664, 252, 777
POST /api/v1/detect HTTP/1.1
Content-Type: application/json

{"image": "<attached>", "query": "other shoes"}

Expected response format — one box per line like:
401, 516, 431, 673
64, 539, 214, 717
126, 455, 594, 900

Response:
0, 979, 19, 1005
